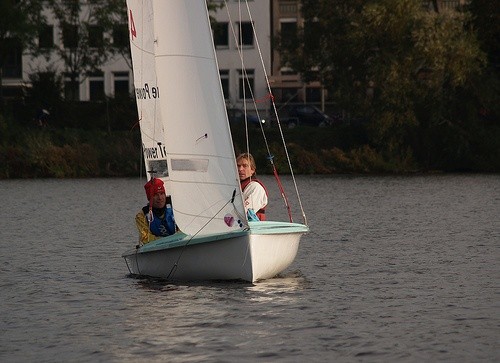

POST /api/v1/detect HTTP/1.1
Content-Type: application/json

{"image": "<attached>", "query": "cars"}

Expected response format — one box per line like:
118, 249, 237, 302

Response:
225, 108, 264, 128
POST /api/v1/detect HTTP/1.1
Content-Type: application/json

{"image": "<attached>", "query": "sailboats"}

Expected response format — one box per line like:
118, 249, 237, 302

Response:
121, 1, 310, 283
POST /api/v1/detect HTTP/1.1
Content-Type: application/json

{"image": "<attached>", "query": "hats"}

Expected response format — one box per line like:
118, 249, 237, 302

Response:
144, 178, 164, 199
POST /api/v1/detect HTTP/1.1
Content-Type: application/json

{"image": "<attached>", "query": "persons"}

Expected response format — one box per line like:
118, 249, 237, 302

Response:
134, 177, 178, 245
235, 152, 268, 222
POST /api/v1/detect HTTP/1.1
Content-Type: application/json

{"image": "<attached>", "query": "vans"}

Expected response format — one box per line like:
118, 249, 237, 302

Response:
272, 104, 334, 129
